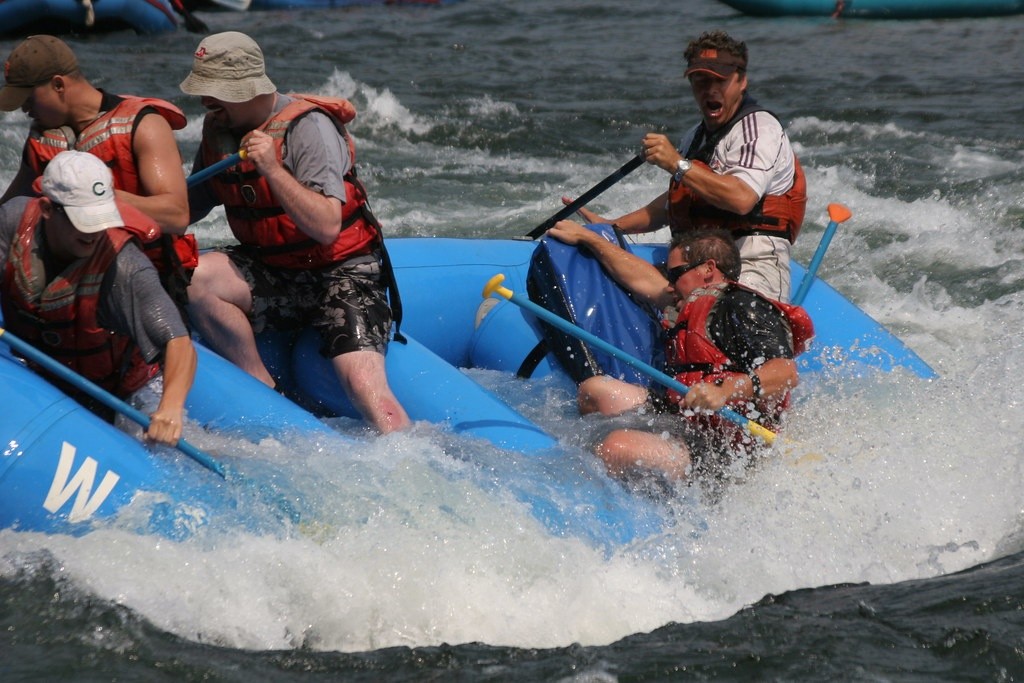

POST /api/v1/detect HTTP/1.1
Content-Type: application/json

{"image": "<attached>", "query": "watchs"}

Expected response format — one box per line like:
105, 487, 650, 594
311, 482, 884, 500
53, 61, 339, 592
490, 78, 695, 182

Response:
674, 157, 691, 184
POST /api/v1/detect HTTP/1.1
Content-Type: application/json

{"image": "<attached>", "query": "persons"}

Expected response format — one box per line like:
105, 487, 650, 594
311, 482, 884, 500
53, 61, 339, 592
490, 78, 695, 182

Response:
561, 29, 796, 302
0, 34, 200, 338
0, 149, 198, 447
544, 219, 814, 506
180, 31, 414, 434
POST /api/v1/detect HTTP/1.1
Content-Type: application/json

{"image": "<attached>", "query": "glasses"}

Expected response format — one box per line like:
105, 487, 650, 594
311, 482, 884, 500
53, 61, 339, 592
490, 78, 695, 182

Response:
666, 256, 739, 285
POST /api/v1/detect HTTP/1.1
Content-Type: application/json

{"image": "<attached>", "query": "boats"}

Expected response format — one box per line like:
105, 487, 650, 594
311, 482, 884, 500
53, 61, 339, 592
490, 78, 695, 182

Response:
716, 0, 1024, 19
0, 234, 943, 566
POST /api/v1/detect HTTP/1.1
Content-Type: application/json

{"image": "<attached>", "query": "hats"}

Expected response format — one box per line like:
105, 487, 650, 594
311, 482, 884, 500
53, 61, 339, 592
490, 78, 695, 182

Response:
0, 35, 78, 112
178, 30, 277, 103
683, 49, 746, 79
41, 149, 125, 233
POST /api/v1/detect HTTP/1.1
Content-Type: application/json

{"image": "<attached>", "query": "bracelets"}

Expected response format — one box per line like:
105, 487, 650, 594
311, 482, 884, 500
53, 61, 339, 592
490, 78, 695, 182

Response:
747, 367, 761, 397
